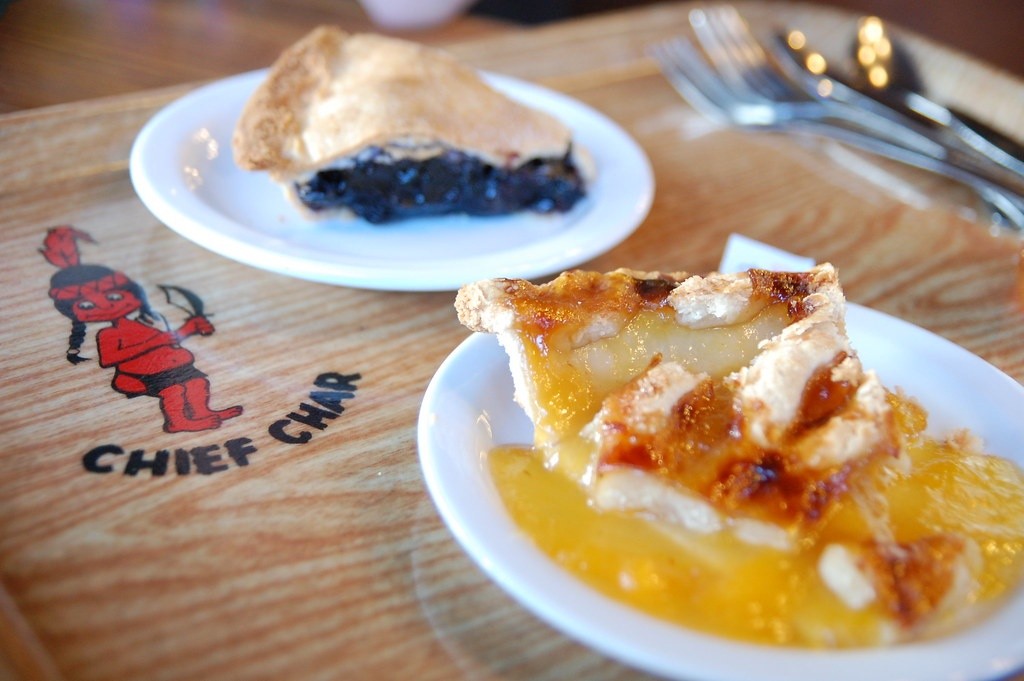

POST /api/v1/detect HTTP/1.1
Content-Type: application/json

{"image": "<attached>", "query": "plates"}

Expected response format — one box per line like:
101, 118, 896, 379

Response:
417, 302, 1024, 680
130, 68, 657, 290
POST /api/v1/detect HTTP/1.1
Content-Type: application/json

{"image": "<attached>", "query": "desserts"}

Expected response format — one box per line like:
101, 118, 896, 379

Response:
453, 264, 983, 635
236, 24, 596, 227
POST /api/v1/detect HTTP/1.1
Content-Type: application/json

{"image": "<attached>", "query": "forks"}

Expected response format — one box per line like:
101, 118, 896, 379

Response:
652, 5, 1024, 239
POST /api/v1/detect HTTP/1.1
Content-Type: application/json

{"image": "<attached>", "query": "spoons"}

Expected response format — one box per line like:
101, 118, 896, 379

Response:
851, 12, 1024, 180
